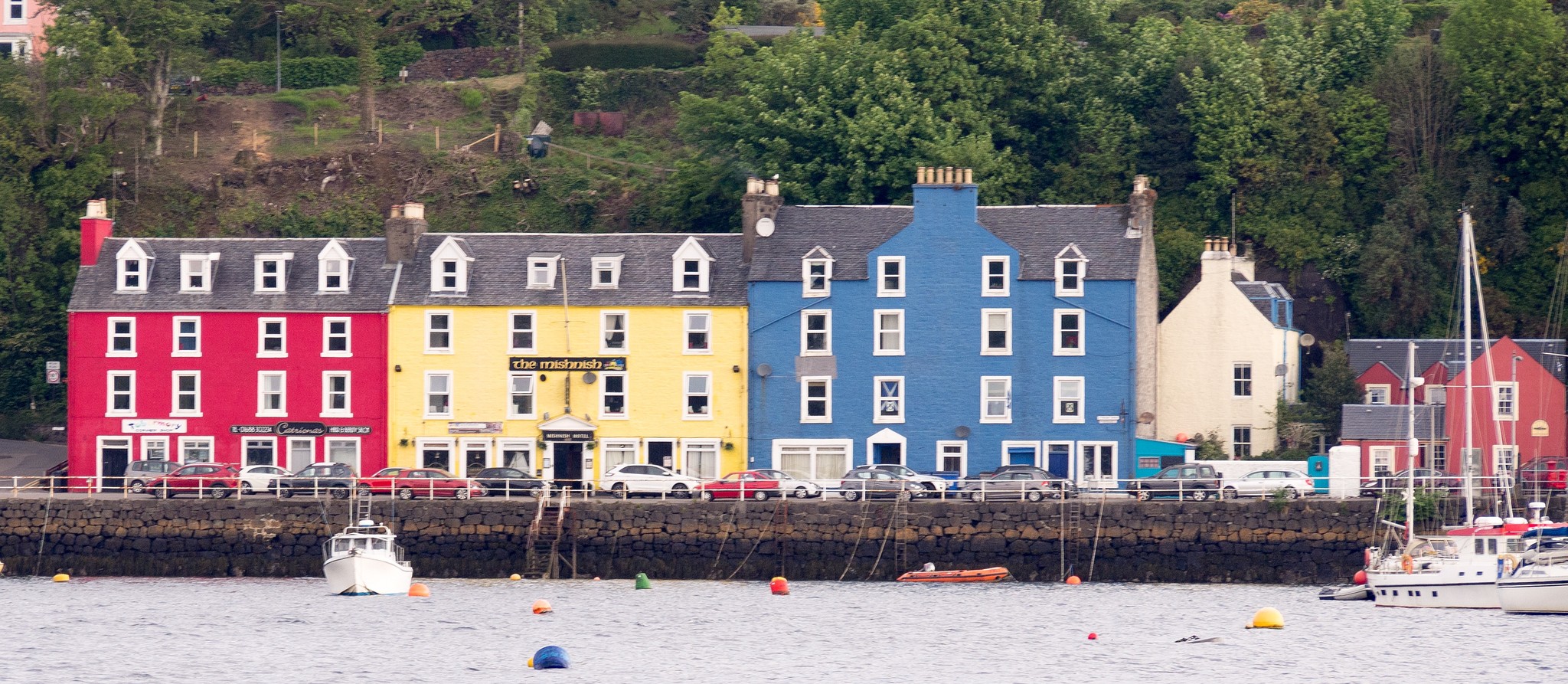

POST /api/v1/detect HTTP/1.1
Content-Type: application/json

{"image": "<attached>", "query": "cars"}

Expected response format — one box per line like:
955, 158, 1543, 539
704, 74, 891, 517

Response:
370, 467, 420, 477
1360, 466, 1461, 499
1217, 468, 1315, 500
692, 471, 781, 502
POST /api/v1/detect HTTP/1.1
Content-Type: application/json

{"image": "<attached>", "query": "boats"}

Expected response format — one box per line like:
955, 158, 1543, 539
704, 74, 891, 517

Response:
321, 483, 413, 596
1317, 583, 1375, 601
896, 566, 1017, 583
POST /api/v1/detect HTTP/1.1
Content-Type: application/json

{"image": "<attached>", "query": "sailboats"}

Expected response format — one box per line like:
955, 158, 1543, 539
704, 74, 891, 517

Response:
1361, 201, 1568, 615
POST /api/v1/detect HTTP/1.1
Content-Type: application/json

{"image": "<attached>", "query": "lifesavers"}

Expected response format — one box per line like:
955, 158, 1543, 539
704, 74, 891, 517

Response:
1401, 554, 1413, 575
1498, 554, 1518, 571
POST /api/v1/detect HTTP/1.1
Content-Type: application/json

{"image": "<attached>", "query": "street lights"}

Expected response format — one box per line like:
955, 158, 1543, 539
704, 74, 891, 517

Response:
1512, 349, 1524, 479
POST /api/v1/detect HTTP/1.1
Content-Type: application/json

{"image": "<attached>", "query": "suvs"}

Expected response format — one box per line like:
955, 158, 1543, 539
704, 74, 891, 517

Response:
1126, 463, 1219, 502
961, 470, 1054, 502
1491, 456, 1568, 496
475, 467, 558, 498
856, 463, 946, 499
741, 469, 821, 498
992, 464, 1079, 499
124, 460, 194, 494
144, 462, 242, 500
839, 469, 928, 502
237, 465, 295, 496
395, 468, 484, 500
599, 463, 701, 499
267, 462, 356, 499
